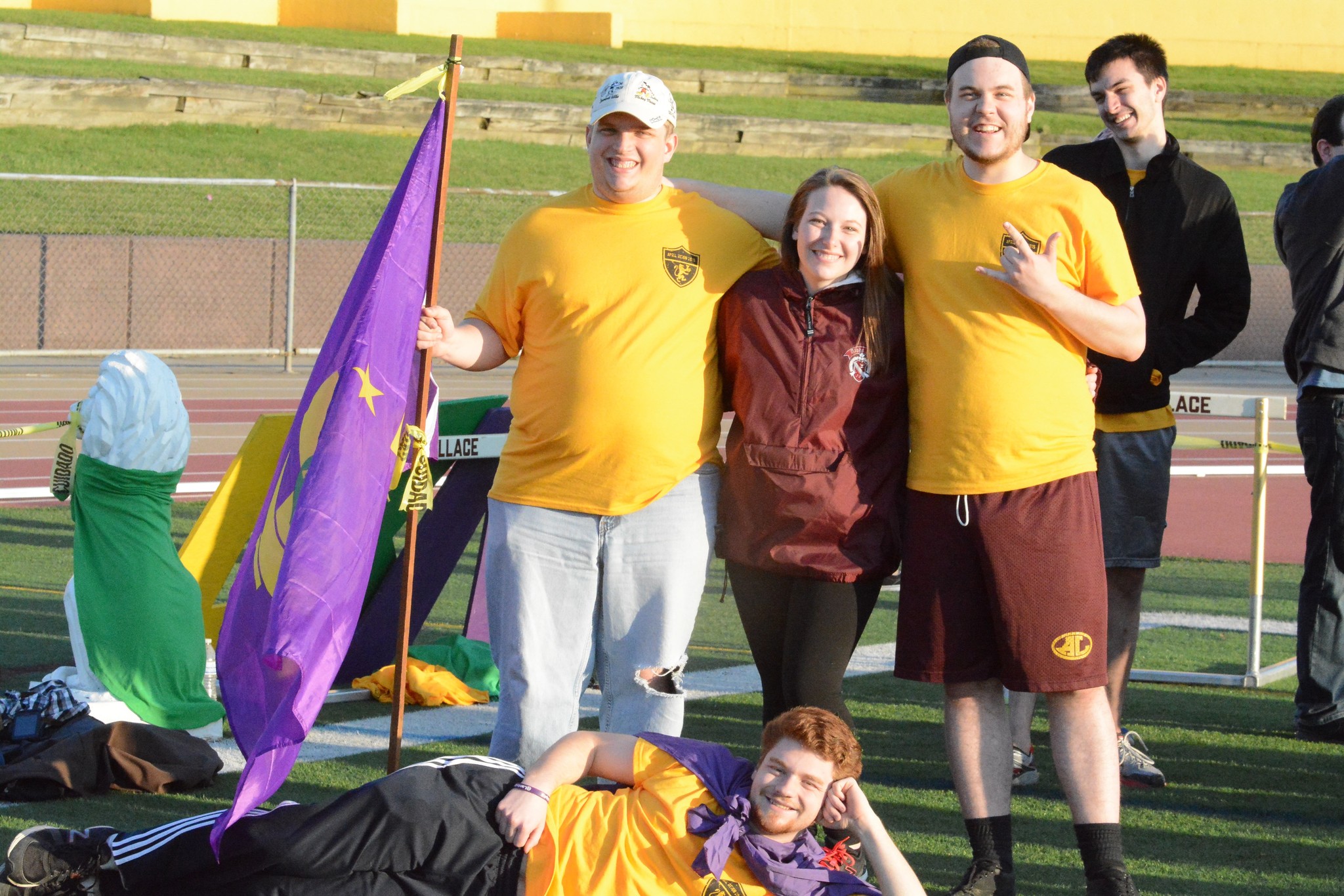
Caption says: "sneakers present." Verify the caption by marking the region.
[951,858,1015,896]
[1012,744,1041,787]
[1118,727,1165,789]
[0,861,99,896]
[818,836,867,881]
[1086,870,1139,896]
[7,825,122,887]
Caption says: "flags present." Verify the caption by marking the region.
[205,94,447,847]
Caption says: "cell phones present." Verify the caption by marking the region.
[11,712,40,741]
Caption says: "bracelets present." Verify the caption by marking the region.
[513,783,551,803]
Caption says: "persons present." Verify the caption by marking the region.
[1006,33,1251,794]
[668,34,1148,895]
[714,166,914,776]
[418,75,781,778]
[0,705,926,896]
[1271,92,1344,744]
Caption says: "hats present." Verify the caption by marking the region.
[947,35,1034,141]
[589,70,678,128]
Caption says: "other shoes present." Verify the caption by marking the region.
[1298,717,1344,744]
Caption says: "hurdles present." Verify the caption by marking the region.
[1127,390,1299,688]
